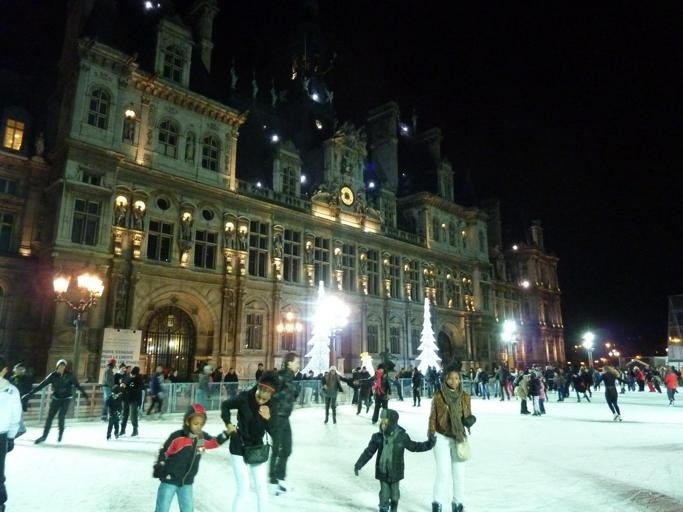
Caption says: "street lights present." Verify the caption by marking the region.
[582,332,593,368]
[578,348,619,372]
[500,319,517,370]
[315,294,349,372]
[275,307,303,354]
[52,264,103,419]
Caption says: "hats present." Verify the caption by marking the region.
[378,408,398,435]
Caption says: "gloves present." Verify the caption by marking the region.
[464,415,476,428]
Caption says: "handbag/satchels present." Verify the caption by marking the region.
[242,444,270,463]
[458,439,470,459]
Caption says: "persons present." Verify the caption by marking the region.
[426,368,474,512]
[195,361,240,400]
[221,354,304,512]
[305,362,441,424]
[102,358,178,441]
[471,362,682,422]
[352,409,438,512]
[1,354,88,511]
[152,403,236,512]
